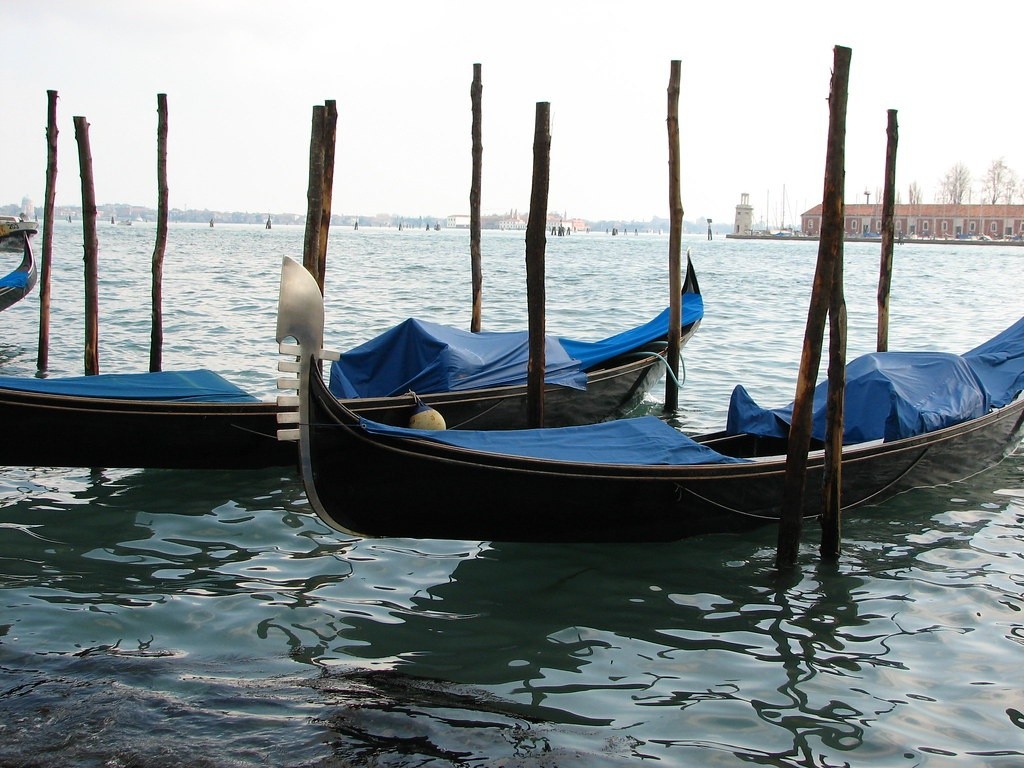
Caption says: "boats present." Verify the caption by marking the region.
[275,252,1024,545]
[0,230,38,312]
[0,213,39,244]
[0,249,708,471]
[117,219,132,225]
